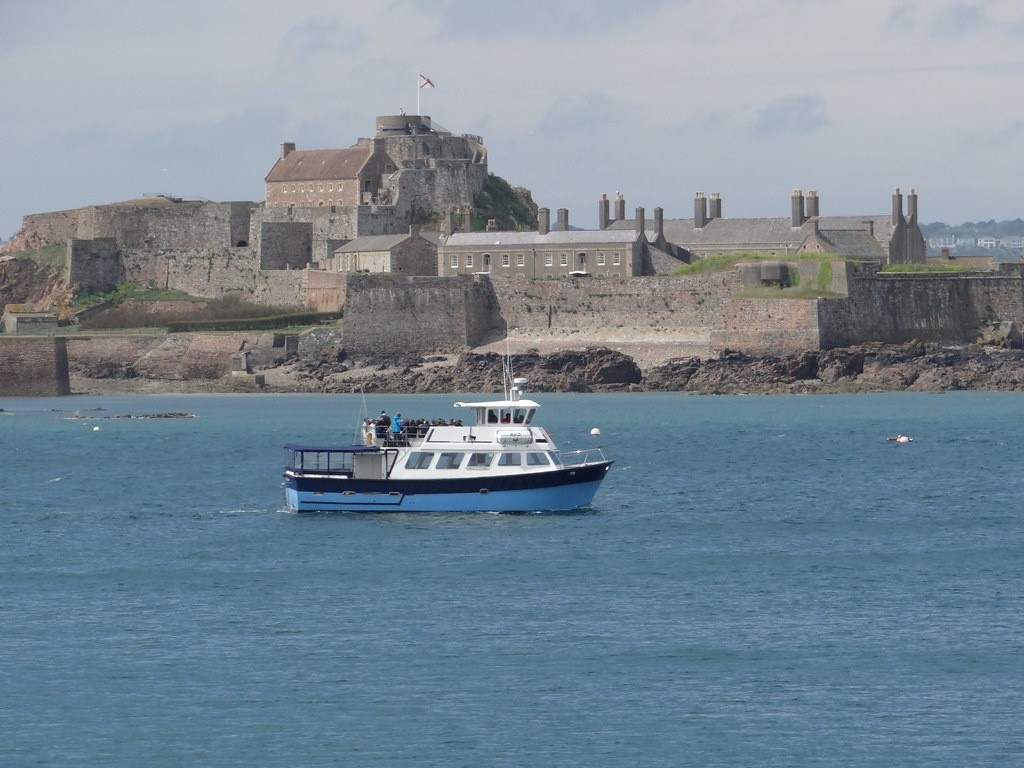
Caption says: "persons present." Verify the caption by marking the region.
[401,417,431,447]
[430,418,448,426]
[374,410,396,447]
[487,410,498,423]
[501,413,510,423]
[392,413,405,446]
[514,414,530,424]
[450,418,462,427]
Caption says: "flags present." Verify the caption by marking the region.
[417,74,435,89]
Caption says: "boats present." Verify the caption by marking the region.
[282,314,617,517]
